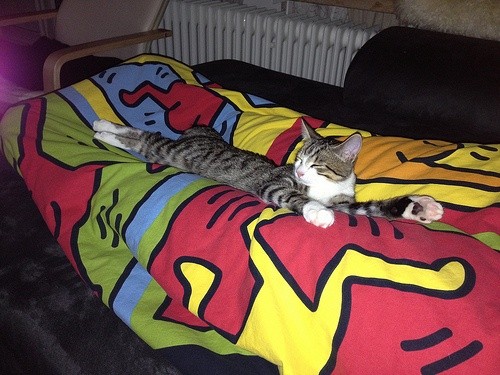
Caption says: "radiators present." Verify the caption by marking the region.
[149,0,379,87]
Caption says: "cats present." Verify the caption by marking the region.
[93,115,444,228]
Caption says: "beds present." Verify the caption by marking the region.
[0,25,500,375]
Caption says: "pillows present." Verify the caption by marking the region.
[393,0,500,40]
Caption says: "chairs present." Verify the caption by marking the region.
[0,1,173,106]
